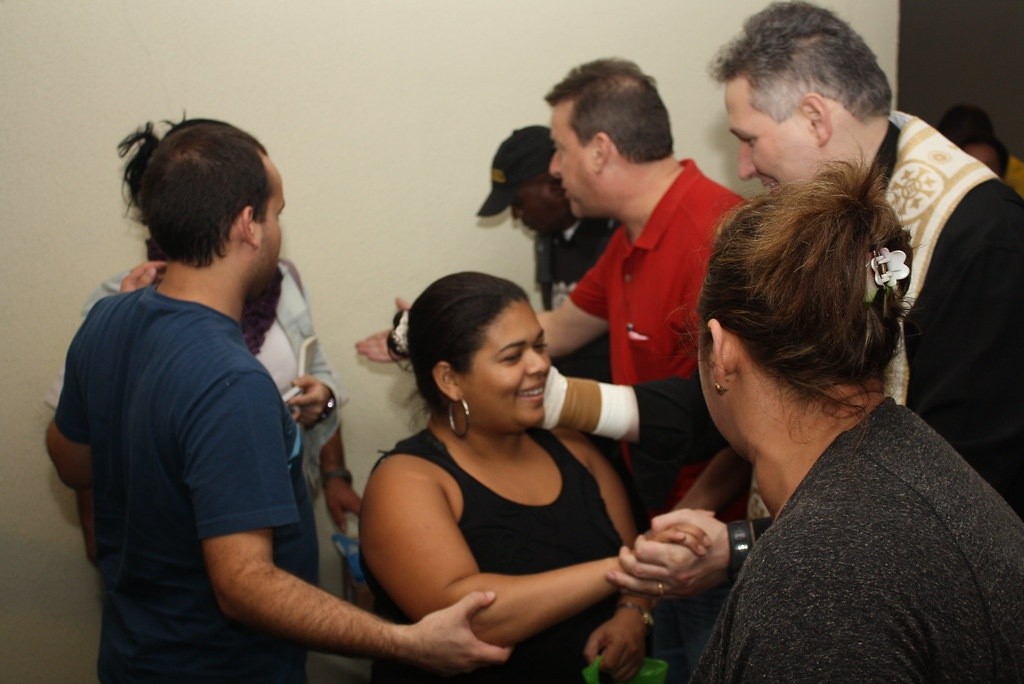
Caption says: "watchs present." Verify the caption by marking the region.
[303,389,336,429]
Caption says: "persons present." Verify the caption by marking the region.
[355,56,751,684]
[942,102,1010,177]
[45,119,514,684]
[389,1,1024,601]
[694,160,1024,684]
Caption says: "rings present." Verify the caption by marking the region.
[657,581,665,595]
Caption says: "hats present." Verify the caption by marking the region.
[477,126,557,217]
[936,105,1010,169]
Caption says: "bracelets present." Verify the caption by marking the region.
[324,469,351,484]
[616,601,654,630]
[726,519,753,585]
